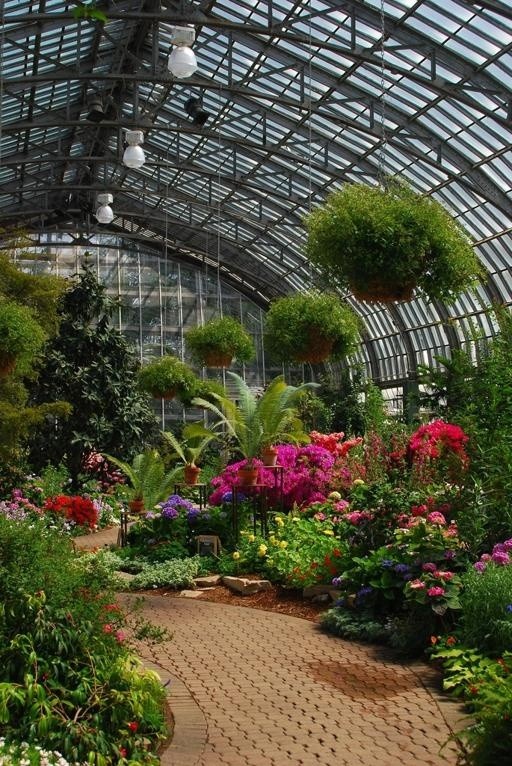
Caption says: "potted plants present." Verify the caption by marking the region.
[307,175,488,314]
[134,317,254,401]
[97,373,321,510]
[260,290,360,368]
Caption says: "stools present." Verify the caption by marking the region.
[119,513,145,548]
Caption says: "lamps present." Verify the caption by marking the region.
[88,24,208,227]
